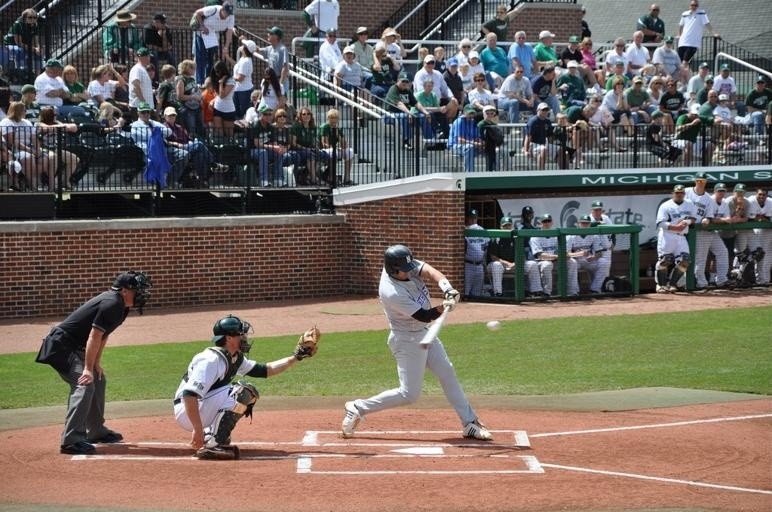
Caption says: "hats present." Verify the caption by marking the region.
[318,26,768,121]
[465,170,754,227]
[20,0,288,118]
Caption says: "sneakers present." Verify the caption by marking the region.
[462,417,493,441]
[655,280,680,296]
[60,440,97,455]
[197,444,236,460]
[7,163,359,192]
[87,430,124,444]
[342,400,362,439]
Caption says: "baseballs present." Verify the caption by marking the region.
[487,321,500,332]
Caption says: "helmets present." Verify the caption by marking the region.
[210,313,249,344]
[384,244,421,274]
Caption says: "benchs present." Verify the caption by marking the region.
[481,257,597,301]
[2,39,772,191]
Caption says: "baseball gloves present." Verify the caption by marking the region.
[296,328,320,360]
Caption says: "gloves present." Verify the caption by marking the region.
[441,298,456,312]
[444,286,461,304]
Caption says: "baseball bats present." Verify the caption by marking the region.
[420,305,451,350]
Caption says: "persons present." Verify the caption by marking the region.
[340,242,490,442]
[172,313,318,460]
[34,265,155,451]
